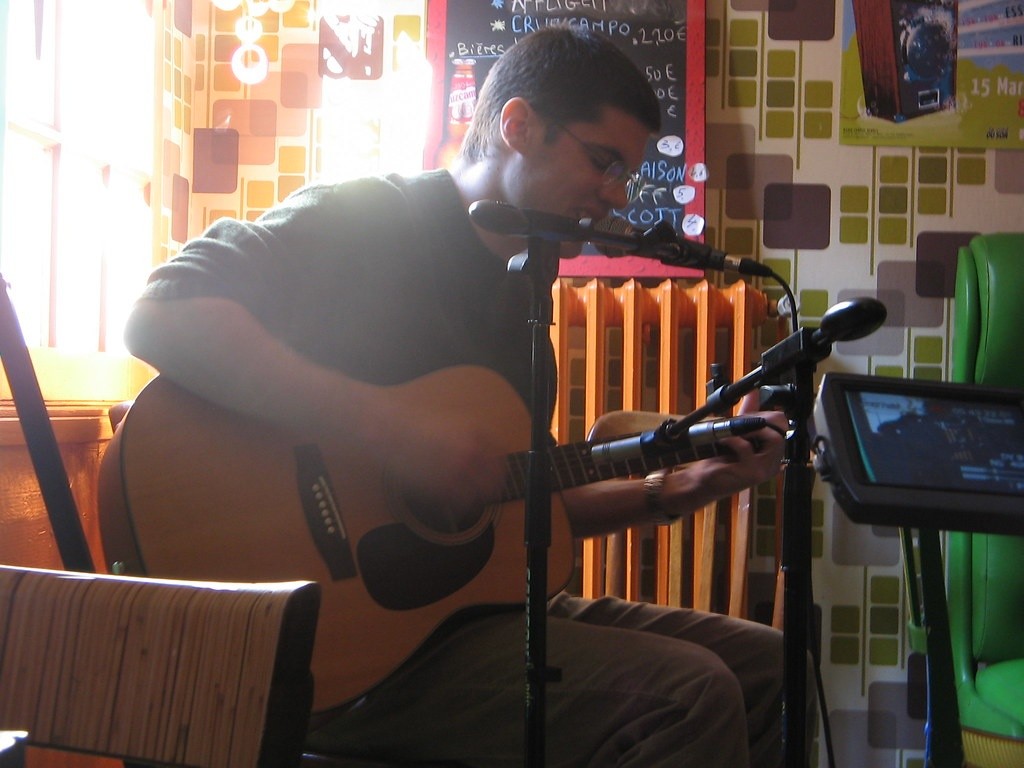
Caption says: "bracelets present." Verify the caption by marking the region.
[644,469,683,526]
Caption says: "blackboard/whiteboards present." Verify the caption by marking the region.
[425,1,705,279]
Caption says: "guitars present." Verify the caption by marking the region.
[93,368,800,715]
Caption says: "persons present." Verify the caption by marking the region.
[127,30,813,768]
[875,399,999,488]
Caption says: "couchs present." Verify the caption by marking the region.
[942,233,1024,768]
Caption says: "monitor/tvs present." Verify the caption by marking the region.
[842,383,1024,496]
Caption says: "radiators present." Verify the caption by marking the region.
[544,276,792,627]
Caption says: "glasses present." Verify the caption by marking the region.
[557,118,645,204]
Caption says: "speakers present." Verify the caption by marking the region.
[851,0,959,124]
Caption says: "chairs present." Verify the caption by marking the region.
[0,406,325,768]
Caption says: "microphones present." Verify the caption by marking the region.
[591,216,772,278]
[590,417,767,465]
